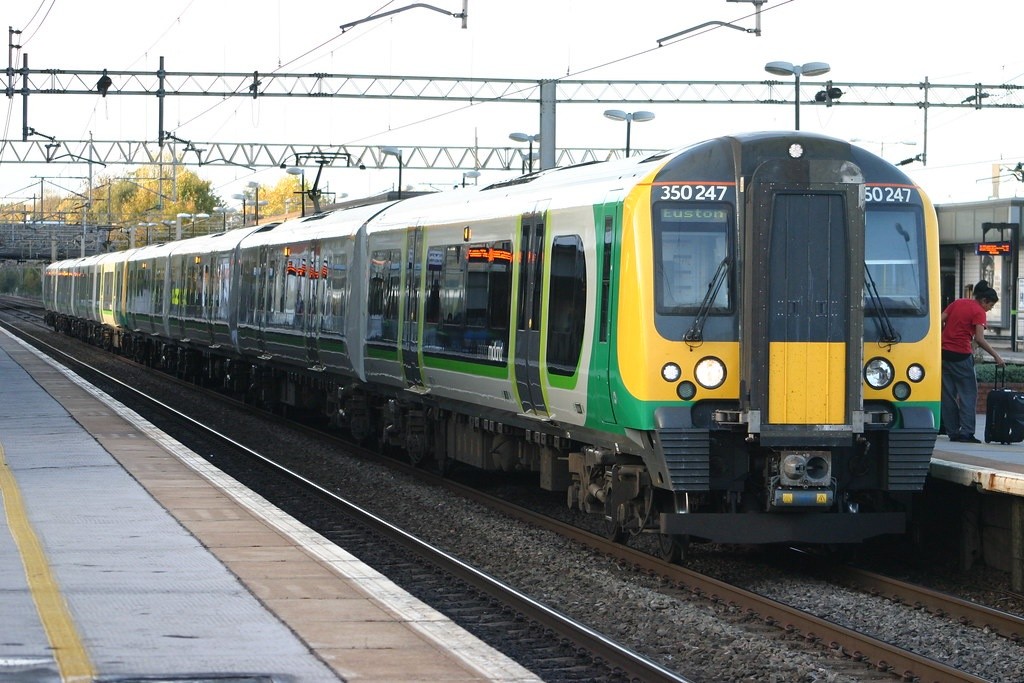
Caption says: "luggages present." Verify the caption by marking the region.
[984,364,1024,445]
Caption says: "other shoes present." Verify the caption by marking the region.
[959,435,981,443]
[950,435,959,441]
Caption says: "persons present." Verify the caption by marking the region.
[941,280,1005,443]
[982,256,994,288]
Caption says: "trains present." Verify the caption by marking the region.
[41,129,943,565]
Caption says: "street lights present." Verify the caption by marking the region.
[160,219,176,241]
[231,193,247,228]
[138,222,158,246]
[120,227,137,249]
[509,132,541,173]
[850,137,917,158]
[212,206,236,232]
[285,167,305,217]
[602,108,656,157]
[893,222,920,297]
[380,145,403,200]
[245,181,259,226]
[177,212,210,237]
[763,60,832,131]
[245,199,269,220]
[462,171,481,188]
